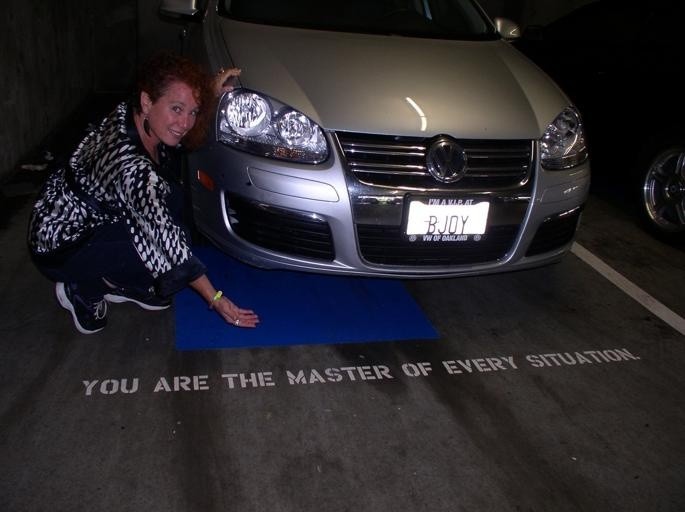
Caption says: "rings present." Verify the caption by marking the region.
[218,68,225,76]
[235,319,240,327]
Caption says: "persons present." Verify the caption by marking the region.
[27,62,261,334]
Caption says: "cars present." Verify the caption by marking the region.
[160,0,593,281]
[510,0,684,250]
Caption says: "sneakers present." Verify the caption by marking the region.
[53,281,107,335]
[103,283,173,311]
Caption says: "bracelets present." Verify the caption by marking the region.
[207,289,224,310]
